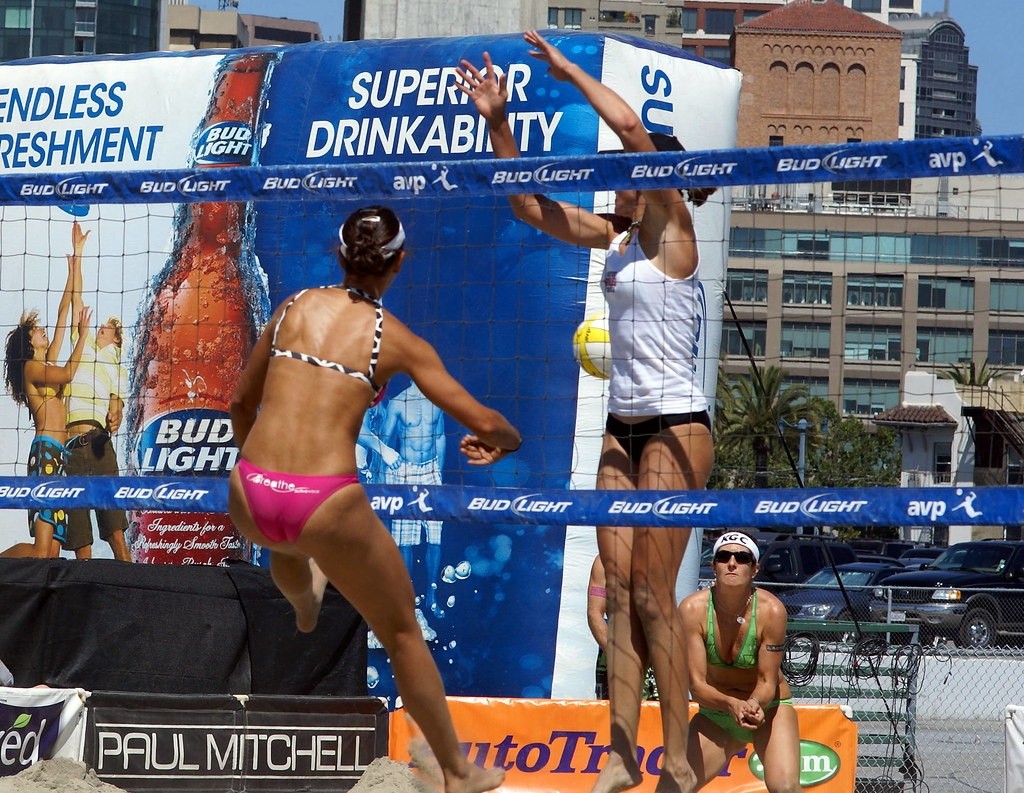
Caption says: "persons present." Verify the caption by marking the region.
[679,531,804,793]
[454,29,716,793]
[229,207,523,793]
[587,555,659,701]
[0,221,131,563]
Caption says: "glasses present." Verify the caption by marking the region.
[714,550,754,565]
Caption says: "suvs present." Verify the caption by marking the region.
[868,537,1024,653]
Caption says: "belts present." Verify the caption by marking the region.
[66,428,103,449]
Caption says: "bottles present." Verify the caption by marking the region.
[131,54,270,565]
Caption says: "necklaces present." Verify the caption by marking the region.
[626,219,645,245]
[717,594,753,617]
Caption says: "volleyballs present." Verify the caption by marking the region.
[573,312,612,378]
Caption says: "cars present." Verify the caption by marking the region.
[699,526,986,625]
[777,561,921,645]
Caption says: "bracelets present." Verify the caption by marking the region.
[501,437,523,453]
